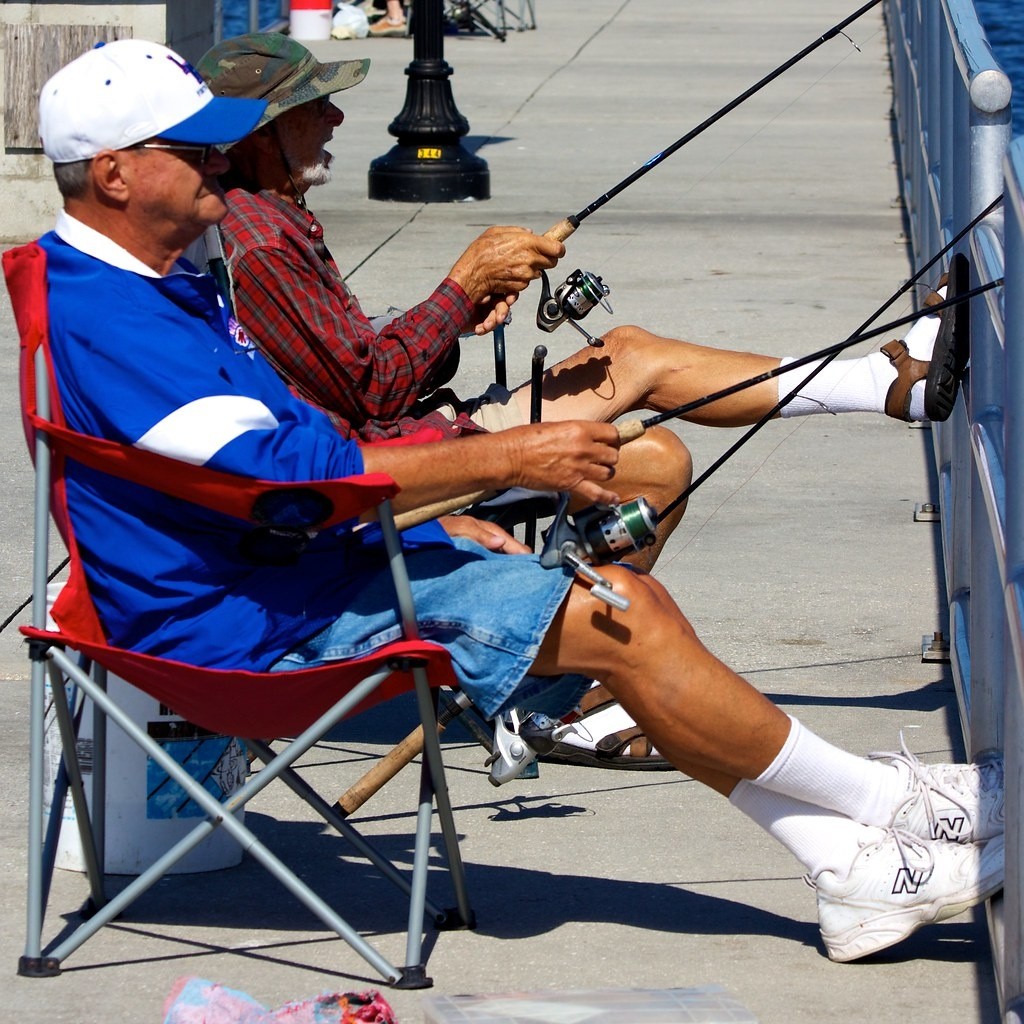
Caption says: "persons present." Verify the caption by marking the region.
[37,38,1008,961]
[192,32,968,768]
[368,0,413,38]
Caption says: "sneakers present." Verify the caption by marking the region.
[808,760,1005,964]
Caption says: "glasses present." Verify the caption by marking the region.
[133,144,214,166]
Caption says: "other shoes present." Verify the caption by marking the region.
[370,16,407,39]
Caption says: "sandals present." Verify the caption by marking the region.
[522,683,675,771]
[880,252,971,425]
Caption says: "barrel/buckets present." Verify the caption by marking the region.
[41,581,247,873]
[289,0,332,39]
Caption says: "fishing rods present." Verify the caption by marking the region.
[535,1,882,348]
[390,274,1000,616]
[330,191,1002,824]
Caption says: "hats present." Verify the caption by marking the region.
[39,40,268,164]
[195,31,373,154]
